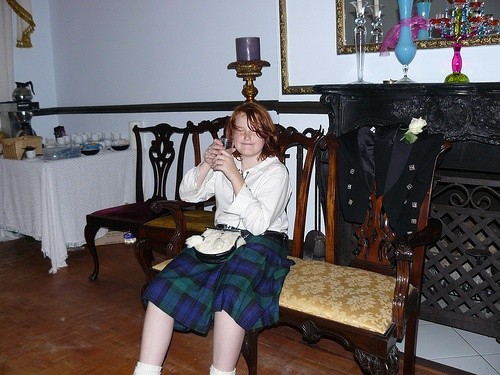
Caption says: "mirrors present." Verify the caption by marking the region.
[335,0,500,55]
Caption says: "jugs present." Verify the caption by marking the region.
[125,120,144,149]
[12,80,36,102]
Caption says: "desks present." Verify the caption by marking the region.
[0,147,154,274]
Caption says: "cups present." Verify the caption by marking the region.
[26,150,36,159]
[219,137,232,155]
[56,131,121,145]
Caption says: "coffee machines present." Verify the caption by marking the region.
[0,101,40,136]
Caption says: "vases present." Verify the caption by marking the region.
[393,0,420,84]
[417,2,431,38]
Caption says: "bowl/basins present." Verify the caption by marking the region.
[81,149,101,156]
[109,145,129,151]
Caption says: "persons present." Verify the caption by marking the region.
[134,103,296,375]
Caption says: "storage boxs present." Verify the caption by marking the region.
[0,133,44,160]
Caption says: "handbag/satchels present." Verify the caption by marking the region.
[186,225,248,264]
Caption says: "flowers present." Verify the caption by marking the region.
[400,116,426,145]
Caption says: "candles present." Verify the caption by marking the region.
[234,36,261,60]
[356,0,362,10]
[374,0,380,13]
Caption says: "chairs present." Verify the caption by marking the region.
[84,116,452,375]
